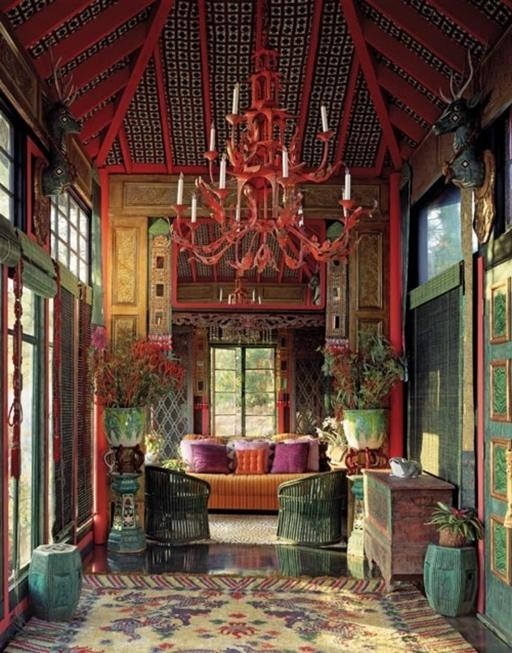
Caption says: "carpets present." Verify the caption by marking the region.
[1,567,478,653]
[143,506,351,553]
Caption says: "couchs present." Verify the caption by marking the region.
[174,432,329,514]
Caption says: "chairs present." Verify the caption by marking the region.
[273,468,353,548]
[144,461,214,547]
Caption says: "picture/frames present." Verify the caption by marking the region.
[489,354,511,427]
[486,274,510,345]
[486,436,512,505]
[485,511,511,588]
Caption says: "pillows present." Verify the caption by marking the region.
[178,432,321,475]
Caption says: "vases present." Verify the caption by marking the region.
[101,404,147,449]
[340,406,390,447]
[329,447,350,464]
[145,451,163,464]
[437,526,466,547]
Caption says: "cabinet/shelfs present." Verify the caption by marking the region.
[357,463,457,600]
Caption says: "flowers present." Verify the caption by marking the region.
[316,414,350,446]
[144,431,166,453]
[84,317,185,406]
[422,499,486,542]
[316,324,407,408]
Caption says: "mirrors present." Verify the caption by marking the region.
[171,214,327,310]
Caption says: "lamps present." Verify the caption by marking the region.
[162,14,371,276]
[215,278,263,304]
